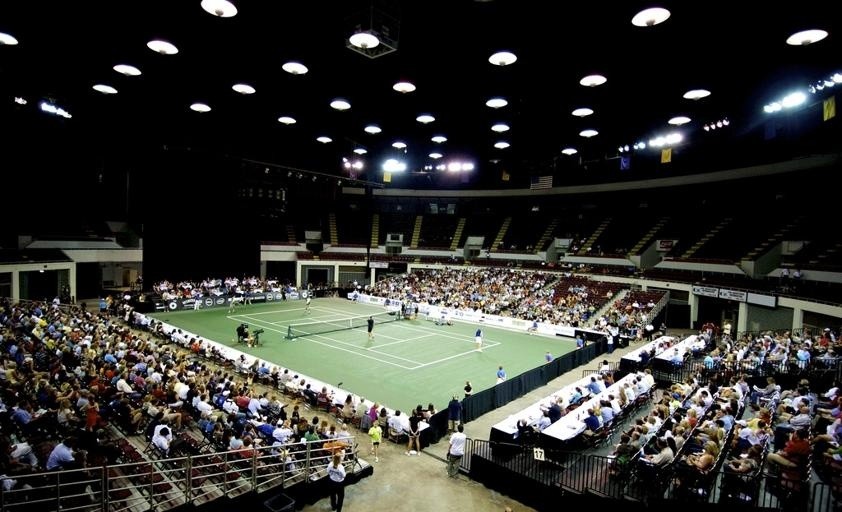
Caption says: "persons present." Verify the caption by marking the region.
[304,295,312,314]
[258,275,348,303]
[0,274,258,512]
[348,239,657,319]
[326,453,346,512]
[496,366,507,385]
[258,360,473,482]
[780,267,792,278]
[474,327,483,353]
[367,316,375,339]
[518,318,842,512]
[792,268,805,278]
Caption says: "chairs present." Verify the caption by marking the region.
[0,269,842,512]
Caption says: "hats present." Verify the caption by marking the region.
[222,389,231,396]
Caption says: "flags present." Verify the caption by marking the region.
[620,156,631,170]
[350,168,358,180]
[501,169,511,181]
[383,171,392,184]
[822,95,839,122]
[661,148,673,164]
[764,117,776,140]
[461,176,469,184]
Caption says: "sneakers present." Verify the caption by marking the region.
[406,450,422,457]
[371,450,380,464]
